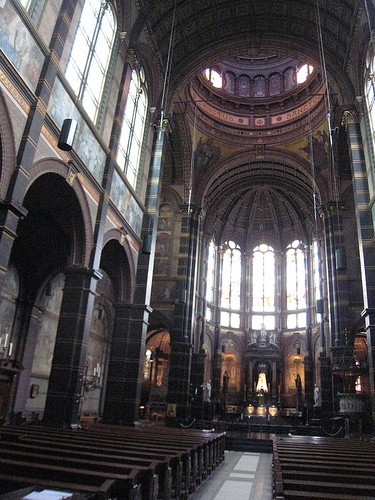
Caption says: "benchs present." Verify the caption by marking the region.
[0,422,375,500]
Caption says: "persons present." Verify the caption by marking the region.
[294,373,304,396]
[206,380,212,403]
[313,384,321,407]
[222,371,229,392]
